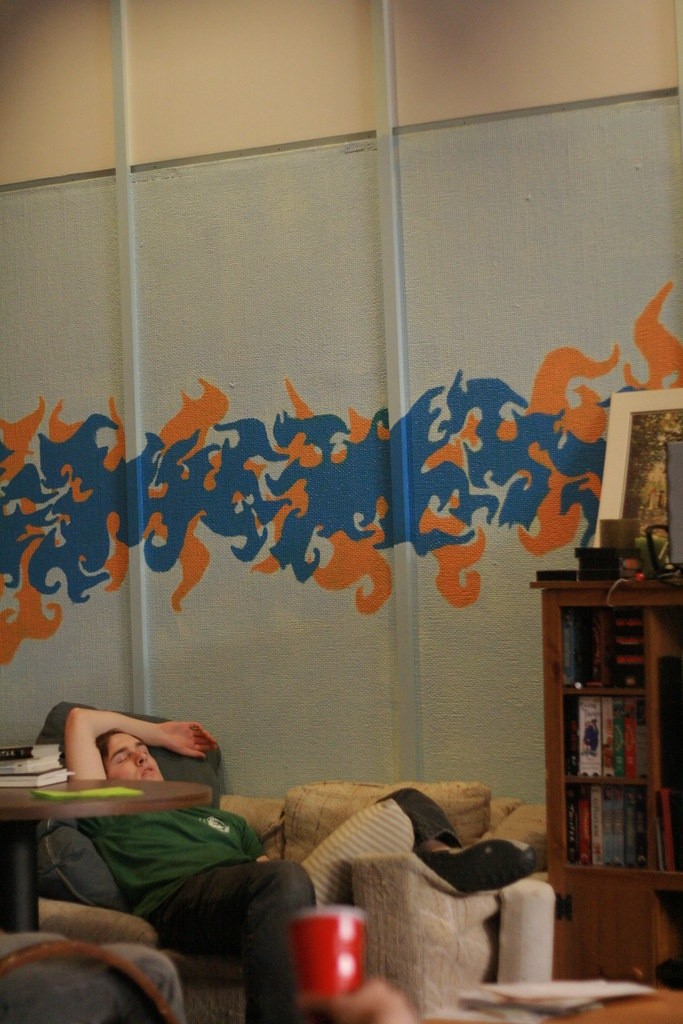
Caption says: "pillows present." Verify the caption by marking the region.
[302,791,448,905]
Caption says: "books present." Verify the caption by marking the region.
[562,607,649,870]
[0,744,67,788]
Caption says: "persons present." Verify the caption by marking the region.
[0,927,186,1024]
[64,707,535,1024]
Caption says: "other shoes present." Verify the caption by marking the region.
[418,838,538,894]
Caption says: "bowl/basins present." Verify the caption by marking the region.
[574,547,640,580]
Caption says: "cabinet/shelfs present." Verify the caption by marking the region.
[529,569,683,992]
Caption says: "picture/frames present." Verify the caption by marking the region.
[591,387,683,570]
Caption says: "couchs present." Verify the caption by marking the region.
[0,778,554,1024]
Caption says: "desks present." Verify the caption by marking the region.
[0,779,215,934]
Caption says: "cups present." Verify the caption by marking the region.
[600,519,640,547]
[636,539,667,577]
[290,905,368,995]
